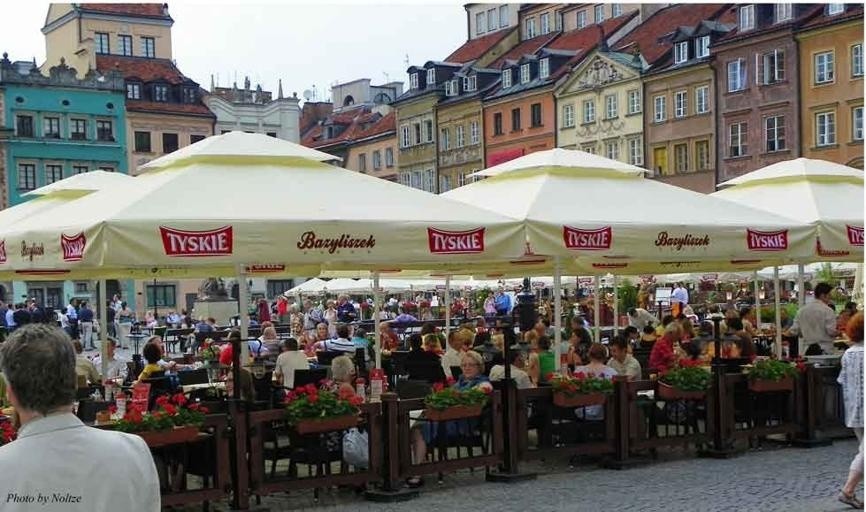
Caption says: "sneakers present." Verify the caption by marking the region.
[837,489,863,509]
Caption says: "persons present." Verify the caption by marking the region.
[165,310,195,328]
[106,293,131,350]
[70,336,197,382]
[191,315,217,354]
[295,294,376,382]
[201,321,310,382]
[1,296,98,351]
[376,285,556,383]
[558,281,862,382]
[248,293,297,323]
[145,310,159,330]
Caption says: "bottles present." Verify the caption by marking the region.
[782,342,790,359]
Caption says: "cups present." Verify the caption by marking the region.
[115,392,126,417]
[355,377,367,405]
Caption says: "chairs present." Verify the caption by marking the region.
[0,280,864,511]
